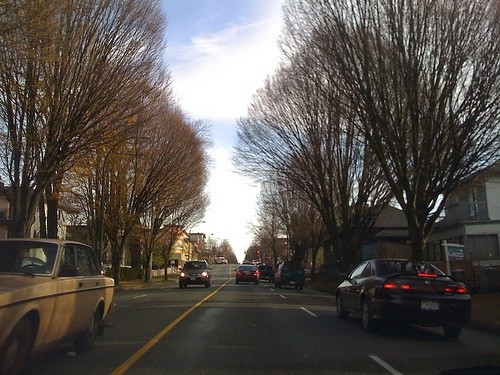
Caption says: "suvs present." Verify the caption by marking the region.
[179,260,212,289]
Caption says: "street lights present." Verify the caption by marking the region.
[188,221,205,260]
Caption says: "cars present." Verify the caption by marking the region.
[216,256,228,264]
[0,238,114,375]
[234,260,305,290]
[335,258,472,339]
